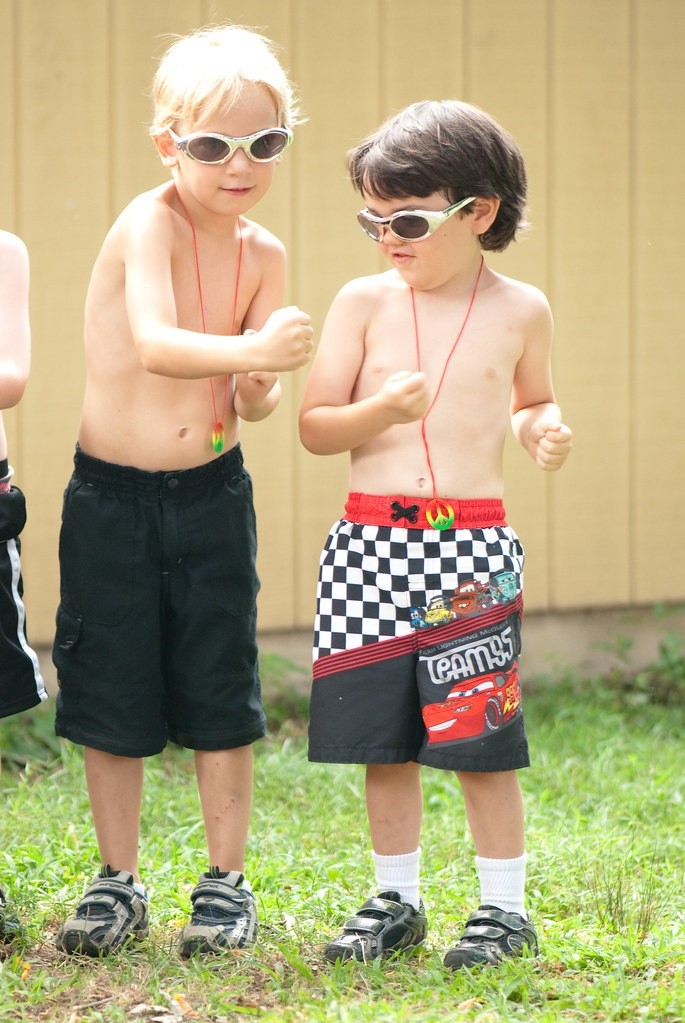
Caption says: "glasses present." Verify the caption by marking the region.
[357,195,476,242]
[167,122,294,165]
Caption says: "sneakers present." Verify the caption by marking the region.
[324,891,428,966]
[177,866,258,957]
[444,905,539,971]
[56,864,149,960]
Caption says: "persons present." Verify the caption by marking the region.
[298,96,573,975]
[51,22,313,965]
[0,226,49,965]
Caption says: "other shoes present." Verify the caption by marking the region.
[0,889,22,962]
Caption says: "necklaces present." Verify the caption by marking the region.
[409,253,485,531]
[171,180,243,453]
